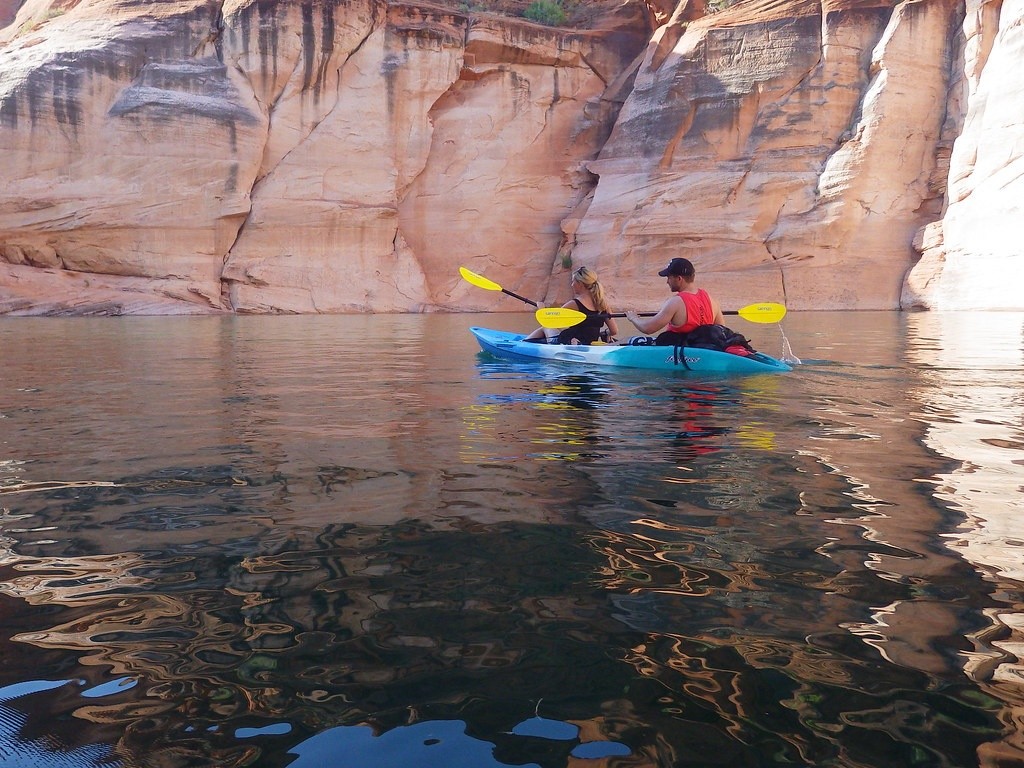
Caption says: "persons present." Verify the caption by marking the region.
[571,258,725,346]
[524,265,618,345]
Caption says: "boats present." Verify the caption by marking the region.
[470,322,796,374]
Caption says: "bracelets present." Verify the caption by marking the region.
[604,328,609,336]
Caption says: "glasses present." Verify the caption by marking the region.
[578,267,585,276]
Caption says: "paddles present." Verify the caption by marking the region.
[534,302,789,330]
[458,266,540,309]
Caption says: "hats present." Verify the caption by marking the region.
[659,258,693,277]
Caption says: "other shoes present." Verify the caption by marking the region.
[568,337,582,345]
